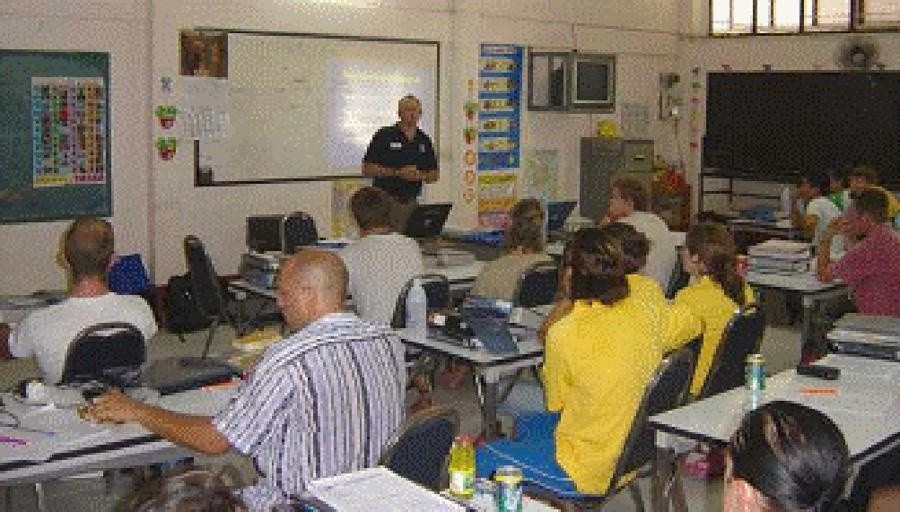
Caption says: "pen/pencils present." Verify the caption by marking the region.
[799,389,836,393]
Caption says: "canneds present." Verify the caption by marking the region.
[741,352,767,389]
[494,464,527,512]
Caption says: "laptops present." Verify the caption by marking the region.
[548,201,577,231]
[401,204,454,237]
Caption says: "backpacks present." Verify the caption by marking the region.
[165,271,213,342]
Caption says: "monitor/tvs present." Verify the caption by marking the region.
[245,212,284,254]
[550,57,615,109]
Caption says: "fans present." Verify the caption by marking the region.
[833,34,886,70]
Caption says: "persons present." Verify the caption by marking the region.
[336,187,424,325]
[609,174,675,291]
[675,225,756,398]
[605,221,666,302]
[0,214,160,383]
[90,249,407,505]
[474,228,702,498]
[469,200,555,301]
[361,95,440,231]
[108,464,252,512]
[723,401,853,511]
[790,165,900,354]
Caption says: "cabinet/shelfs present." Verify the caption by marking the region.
[579,137,655,226]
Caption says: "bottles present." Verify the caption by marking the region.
[449,432,476,501]
[407,276,427,335]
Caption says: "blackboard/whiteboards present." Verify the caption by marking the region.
[704,70,900,191]
[193,27,441,188]
